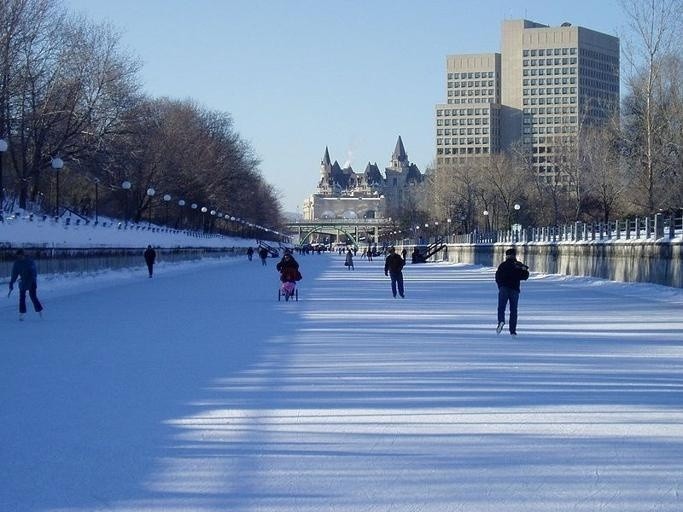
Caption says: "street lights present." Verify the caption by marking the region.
[390,210,489,239]
[0,139,8,206]
[52,155,63,220]
[121,181,294,244]
[514,202,521,224]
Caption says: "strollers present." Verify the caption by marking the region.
[279,266,299,302]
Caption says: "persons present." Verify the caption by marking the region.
[268,243,384,262]
[344,249,354,271]
[245,246,254,262]
[493,247,529,336]
[276,253,302,300]
[412,246,418,264]
[384,247,405,299]
[8,246,43,321]
[258,247,268,266]
[143,244,156,278]
[400,247,407,260]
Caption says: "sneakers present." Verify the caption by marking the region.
[496,321,504,333]
[510,332,517,335]
